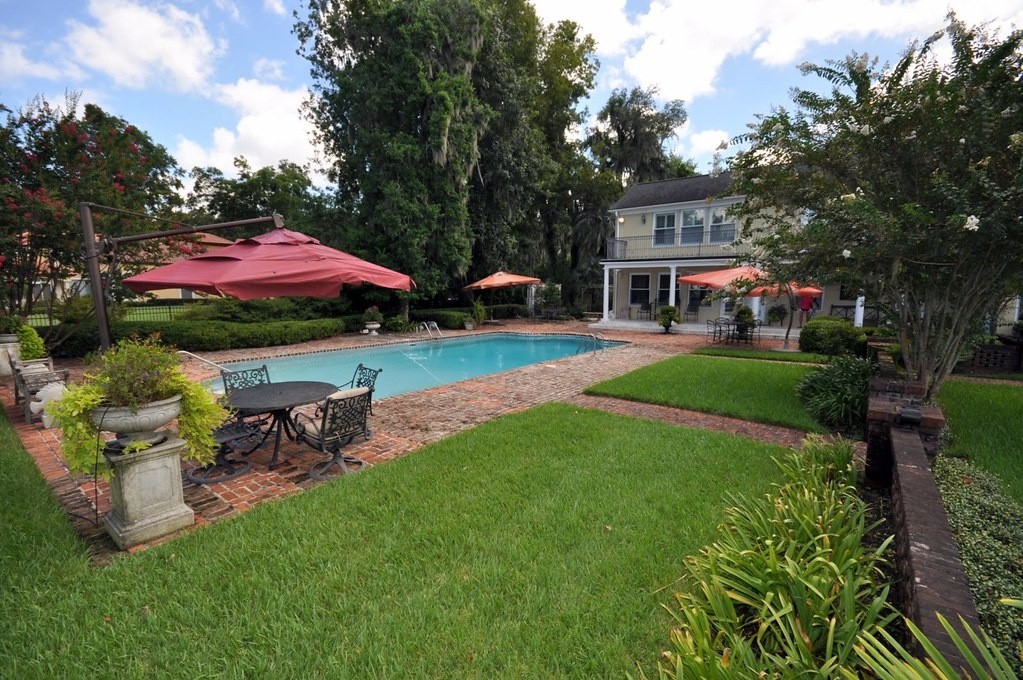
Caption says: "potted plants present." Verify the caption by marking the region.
[463,294,487,330]
[0,314,26,343]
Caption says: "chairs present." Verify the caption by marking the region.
[707,317,762,346]
[186,362,383,483]
[637,303,650,321]
[684,304,699,323]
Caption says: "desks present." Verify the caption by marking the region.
[224,381,339,469]
[724,322,745,344]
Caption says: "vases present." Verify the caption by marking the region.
[365,322,380,336]
[92,393,184,447]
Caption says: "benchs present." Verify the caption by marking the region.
[830,304,879,328]
[649,298,680,323]
[6,346,69,424]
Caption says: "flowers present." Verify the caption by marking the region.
[46,332,240,484]
[361,306,384,324]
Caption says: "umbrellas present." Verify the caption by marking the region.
[122,227,417,301]
[464,271,541,292]
[679,265,824,298]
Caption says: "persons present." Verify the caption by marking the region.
[797,295,817,328]
